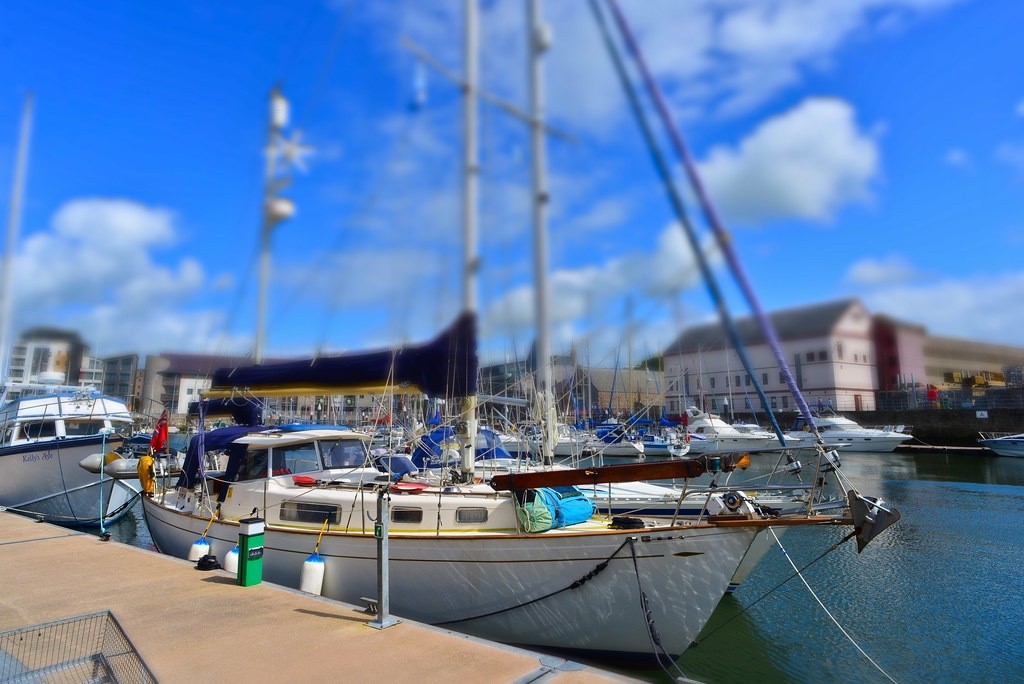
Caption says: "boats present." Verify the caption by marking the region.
[446,401,913,458]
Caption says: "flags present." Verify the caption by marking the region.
[150,410,167,454]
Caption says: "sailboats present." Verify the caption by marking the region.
[141,1,794,664]
[1,383,143,532]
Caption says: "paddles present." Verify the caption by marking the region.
[290,474,430,493]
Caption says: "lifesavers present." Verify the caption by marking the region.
[139,456,156,493]
